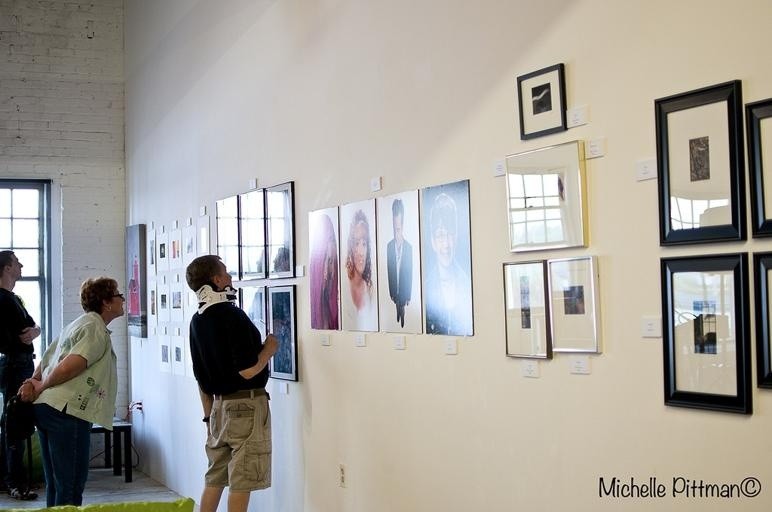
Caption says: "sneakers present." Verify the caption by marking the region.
[7,487,38,500]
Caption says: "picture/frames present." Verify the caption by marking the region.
[241,285,266,347]
[238,188,265,281]
[231,286,241,307]
[504,139,589,255]
[660,251,755,418]
[652,78,748,248]
[517,62,567,141]
[502,259,553,362]
[266,284,299,382]
[744,98,772,239]
[754,251,772,392]
[264,181,296,280]
[215,194,239,282]
[549,255,602,359]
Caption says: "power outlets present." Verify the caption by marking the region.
[136,401,144,413]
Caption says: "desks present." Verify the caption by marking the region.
[91,414,133,483]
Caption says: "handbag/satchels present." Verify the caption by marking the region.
[4,395,35,449]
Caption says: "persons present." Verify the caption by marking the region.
[311,214,339,331]
[186,257,279,511]
[341,210,378,332]
[0,251,41,499]
[387,198,413,327]
[18,277,126,506]
[424,194,471,335]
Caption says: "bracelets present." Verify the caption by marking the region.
[31,376,40,381]
[203,418,212,424]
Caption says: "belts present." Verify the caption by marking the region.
[211,388,267,400]
[0,354,37,359]
